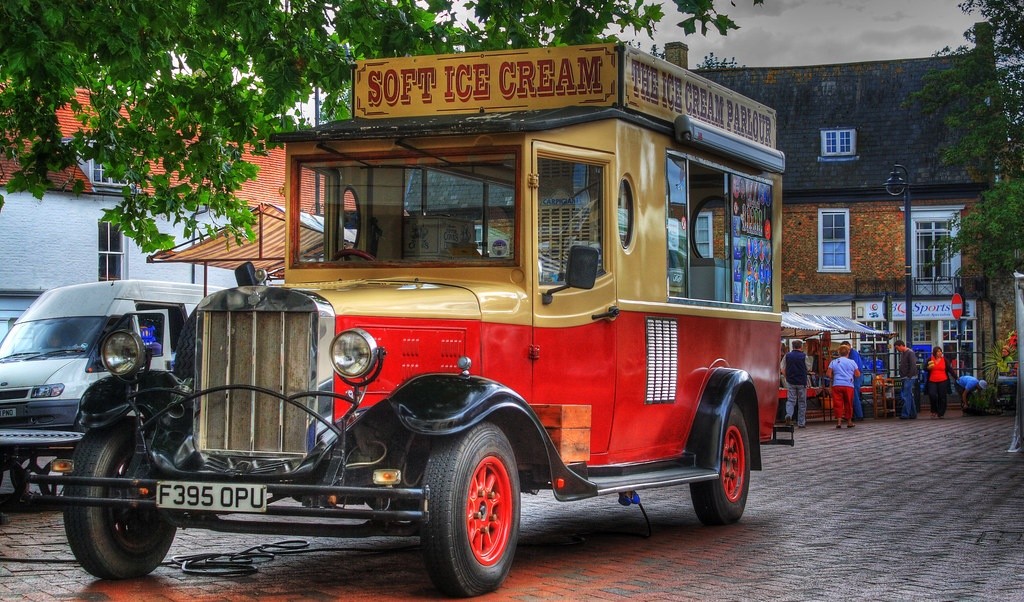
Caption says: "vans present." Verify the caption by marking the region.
[0,280,229,498]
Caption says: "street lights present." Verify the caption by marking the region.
[880,164,914,349]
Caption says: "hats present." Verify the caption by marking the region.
[978,380,988,389]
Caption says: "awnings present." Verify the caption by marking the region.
[780,312,897,339]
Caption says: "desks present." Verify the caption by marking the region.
[779,387,832,423]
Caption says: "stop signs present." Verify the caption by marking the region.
[951,293,964,320]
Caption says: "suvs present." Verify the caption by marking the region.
[21,40,796,602]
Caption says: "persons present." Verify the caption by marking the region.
[997,345,1014,375]
[954,376,987,417]
[923,346,960,419]
[894,340,917,420]
[826,341,864,428]
[780,340,812,428]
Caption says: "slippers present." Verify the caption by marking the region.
[836,423,841,428]
[847,423,855,428]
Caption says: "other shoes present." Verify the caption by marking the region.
[851,416,864,421]
[909,415,917,419]
[899,415,909,419]
[938,414,944,419]
[785,415,791,426]
[931,412,936,419]
[798,424,806,428]
[963,412,968,417]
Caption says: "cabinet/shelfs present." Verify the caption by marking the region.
[402,217,476,261]
[873,378,896,418]
[689,258,726,302]
